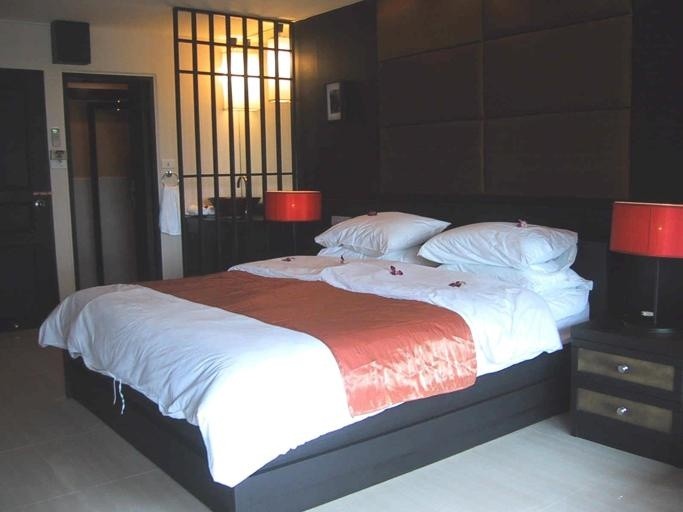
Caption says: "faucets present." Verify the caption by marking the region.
[236,176,247,189]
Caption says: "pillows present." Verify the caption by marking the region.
[314,212,591,292]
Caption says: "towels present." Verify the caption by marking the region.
[157,182,182,236]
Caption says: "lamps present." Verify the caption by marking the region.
[608,200,683,333]
[263,190,322,256]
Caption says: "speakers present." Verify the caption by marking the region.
[50,21,90,65]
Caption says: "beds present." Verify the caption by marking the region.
[61,194,615,512]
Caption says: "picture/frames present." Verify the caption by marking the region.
[323,80,344,122]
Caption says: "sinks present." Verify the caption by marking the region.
[208,196,261,215]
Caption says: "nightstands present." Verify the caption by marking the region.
[570,313,682,469]
